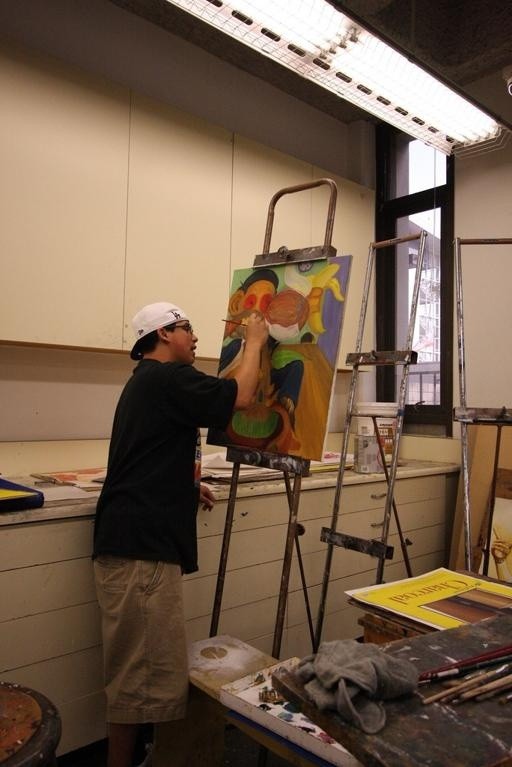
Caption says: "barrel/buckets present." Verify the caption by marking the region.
[356,399,401,473]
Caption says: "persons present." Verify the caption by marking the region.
[91,302,270,767]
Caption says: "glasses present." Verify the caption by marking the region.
[163,323,190,332]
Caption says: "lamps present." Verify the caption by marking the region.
[162,0,512,161]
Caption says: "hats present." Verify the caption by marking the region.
[130,302,189,360]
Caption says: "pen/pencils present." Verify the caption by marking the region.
[419,645,512,705]
[221,319,248,326]
